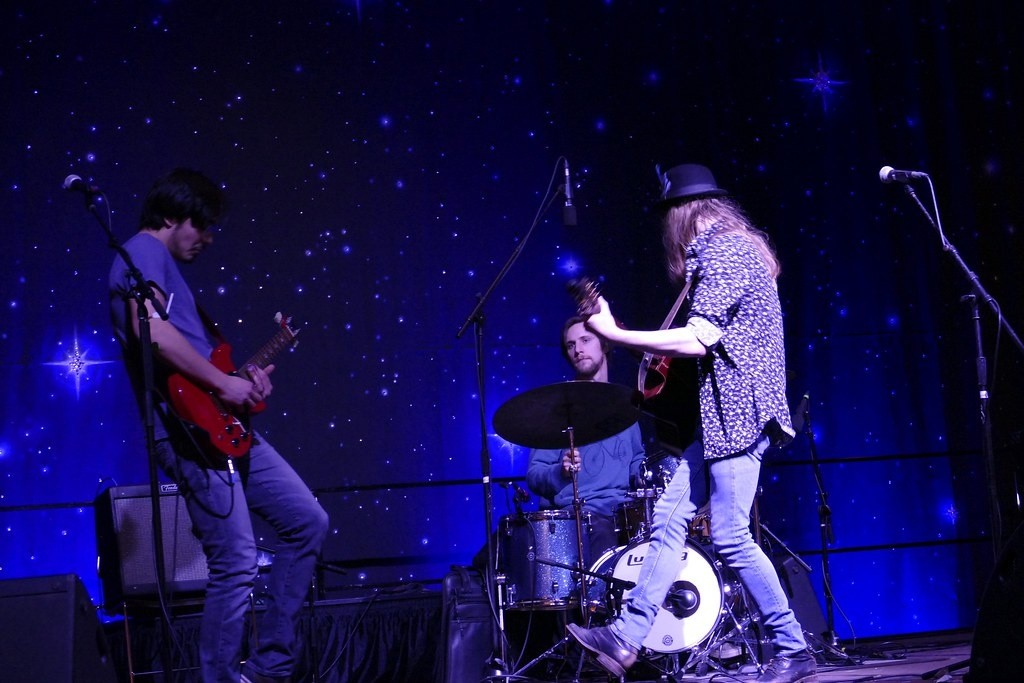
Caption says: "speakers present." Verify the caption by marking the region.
[772,554,832,650]
[0,573,118,683]
[94,481,211,609]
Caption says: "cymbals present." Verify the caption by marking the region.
[491,376,643,448]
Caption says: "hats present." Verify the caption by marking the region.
[652,164,729,206]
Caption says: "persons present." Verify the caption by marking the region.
[524,315,652,619]
[103,168,331,683]
[567,160,819,683]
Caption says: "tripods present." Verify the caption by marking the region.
[505,424,678,683]
[674,412,906,683]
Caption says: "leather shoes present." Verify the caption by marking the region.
[757,649,818,683]
[564,622,638,678]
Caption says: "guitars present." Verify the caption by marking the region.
[564,275,697,413]
[165,311,300,472]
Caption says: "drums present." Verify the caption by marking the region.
[498,505,619,615]
[612,494,661,545]
[584,533,727,655]
[638,448,684,488]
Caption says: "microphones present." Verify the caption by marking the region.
[513,484,530,499]
[879,166,928,184]
[562,159,572,207]
[64,174,102,196]
[792,393,811,433]
